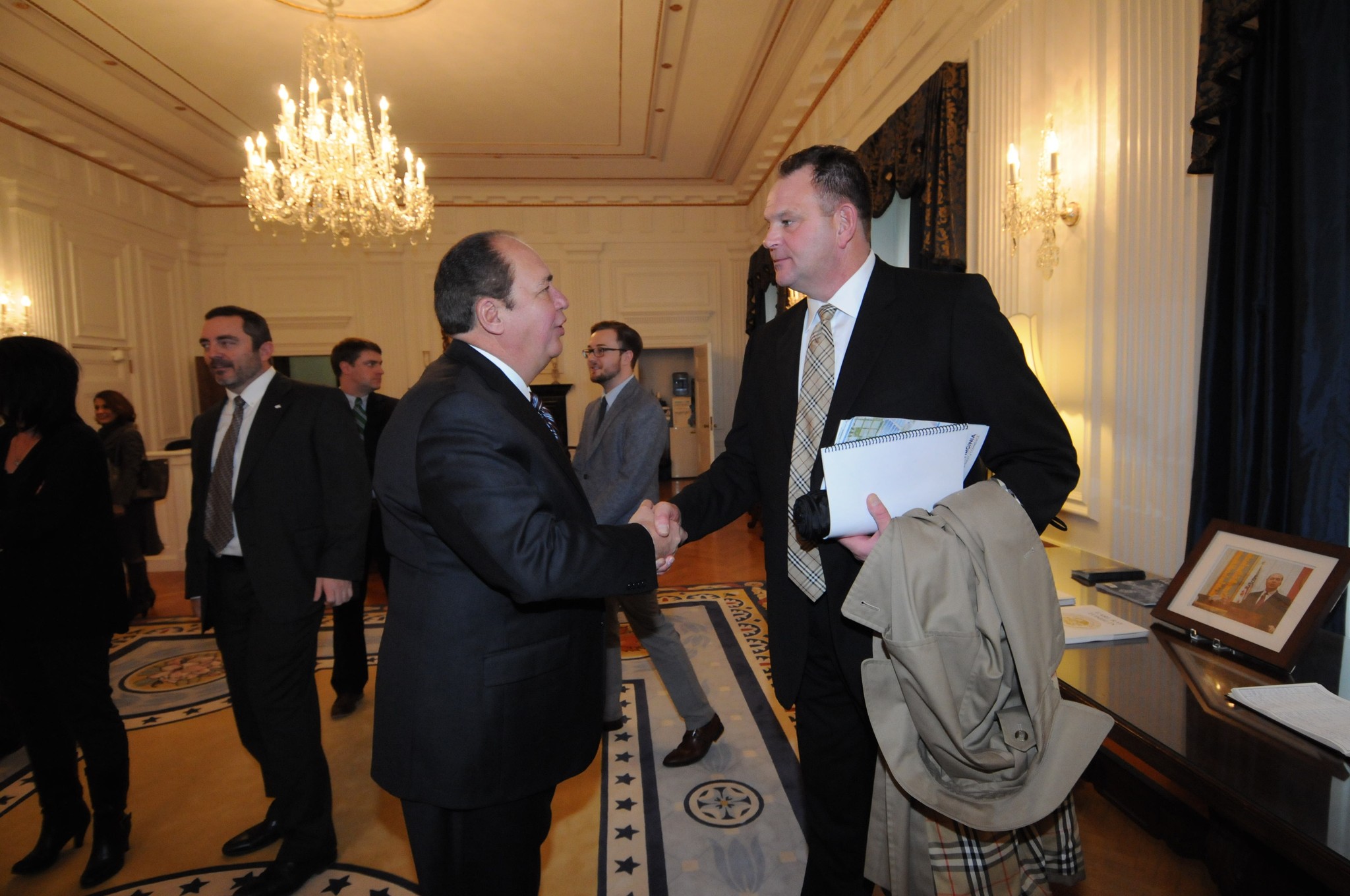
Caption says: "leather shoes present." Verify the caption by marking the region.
[222,819,289,857]
[233,849,337,895]
[330,690,364,719]
[663,712,724,767]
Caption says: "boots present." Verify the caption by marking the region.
[80,757,132,890]
[11,752,91,877]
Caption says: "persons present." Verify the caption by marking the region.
[93,388,164,633]
[0,336,133,890]
[369,227,687,895]
[649,145,1082,894]
[186,303,358,896]
[328,336,400,719]
[569,322,725,766]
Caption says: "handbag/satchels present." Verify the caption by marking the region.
[133,458,170,504]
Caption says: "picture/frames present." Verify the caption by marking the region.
[1148,513,1350,672]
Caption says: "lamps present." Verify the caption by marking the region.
[0,290,34,336]
[240,0,434,251]
[998,113,1081,280]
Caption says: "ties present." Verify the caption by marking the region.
[204,396,246,554]
[351,396,368,441]
[529,391,571,459]
[787,304,837,604]
[593,395,608,438]
[1255,592,1269,611]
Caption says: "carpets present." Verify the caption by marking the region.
[1,579,809,896]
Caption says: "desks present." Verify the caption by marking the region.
[1030,542,1349,896]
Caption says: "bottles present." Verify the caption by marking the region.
[672,371,689,396]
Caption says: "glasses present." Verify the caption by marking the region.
[582,347,627,359]
